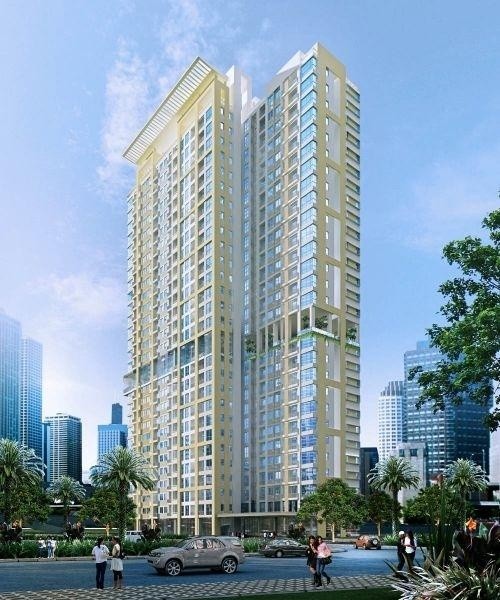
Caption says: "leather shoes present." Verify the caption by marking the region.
[112,586,122,589]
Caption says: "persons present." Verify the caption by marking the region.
[305,535,318,585]
[39,535,57,559]
[110,536,123,589]
[92,538,110,589]
[404,531,417,573]
[316,534,332,588]
[397,530,405,571]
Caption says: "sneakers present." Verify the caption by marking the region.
[97,585,104,589]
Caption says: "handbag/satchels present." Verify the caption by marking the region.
[102,551,109,558]
[319,557,332,565]
[118,553,126,560]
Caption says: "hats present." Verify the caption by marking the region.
[398,531,405,535]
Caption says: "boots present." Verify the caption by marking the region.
[311,572,321,587]
[323,574,331,585]
